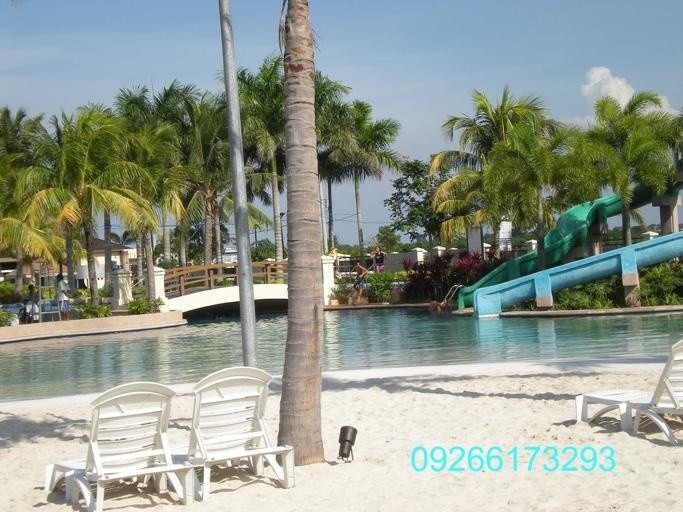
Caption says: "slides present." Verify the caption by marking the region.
[457,159,683,320]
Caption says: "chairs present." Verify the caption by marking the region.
[576,339,683,446]
[171,366,295,502]
[46,381,194,512]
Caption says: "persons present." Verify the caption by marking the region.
[55,273,71,320]
[21,284,39,323]
[348,261,368,305]
[369,245,388,272]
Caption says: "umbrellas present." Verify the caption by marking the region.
[5,255,69,279]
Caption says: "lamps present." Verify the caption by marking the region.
[337,426,358,462]
